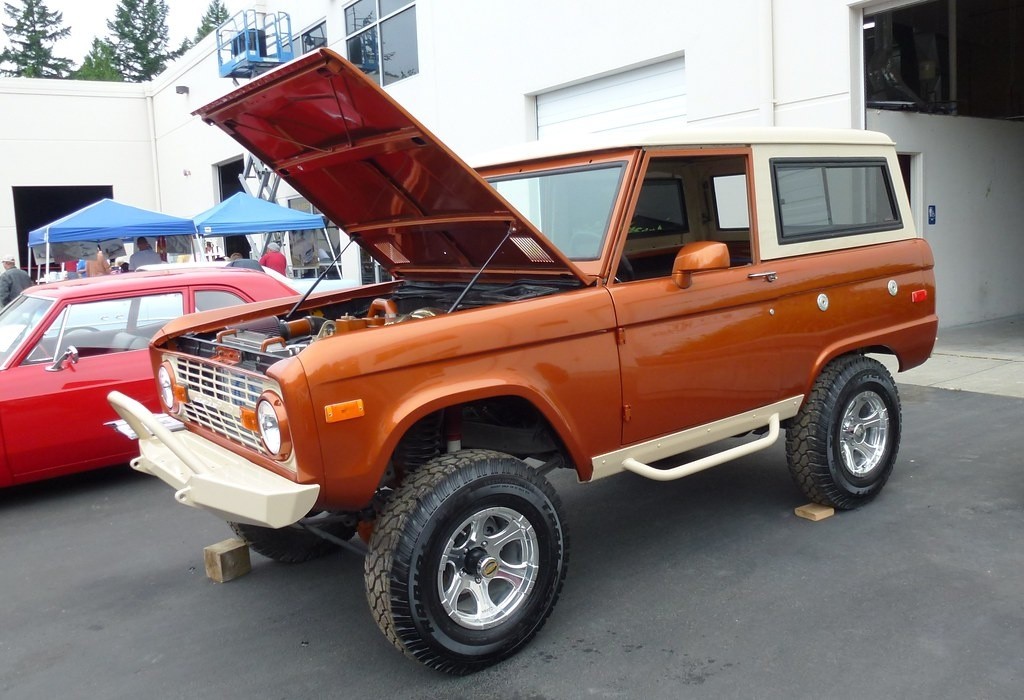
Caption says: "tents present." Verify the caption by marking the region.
[190,191,343,281]
[27,197,206,286]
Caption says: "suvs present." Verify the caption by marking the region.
[103,48,937,680]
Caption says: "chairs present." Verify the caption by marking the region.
[107,333,134,353]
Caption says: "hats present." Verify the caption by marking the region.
[267,242,280,252]
[1,256,15,263]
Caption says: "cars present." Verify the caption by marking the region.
[290,232,316,264]
[130,259,359,296]
[0,268,303,489]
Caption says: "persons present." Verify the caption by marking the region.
[0,255,34,312]
[65,236,163,279]
[224,242,288,278]
